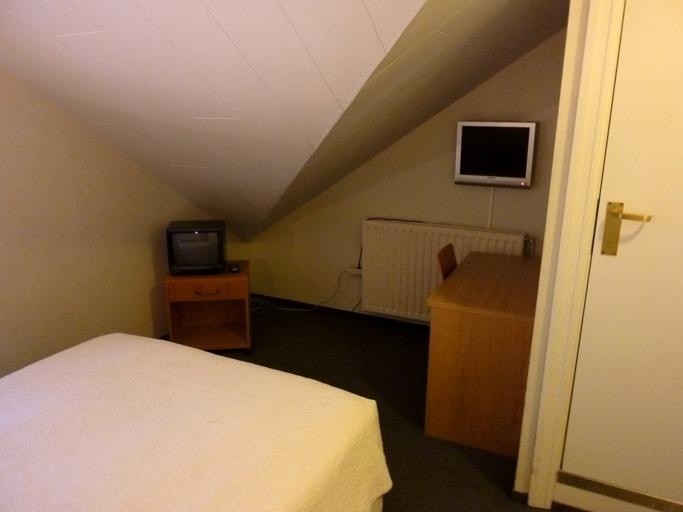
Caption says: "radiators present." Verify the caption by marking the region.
[361,217,535,323]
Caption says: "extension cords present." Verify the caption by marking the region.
[346,268,361,275]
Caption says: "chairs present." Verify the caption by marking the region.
[436,242,458,280]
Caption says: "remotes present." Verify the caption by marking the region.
[230,263,239,273]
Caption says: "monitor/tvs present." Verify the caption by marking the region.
[454,121,537,189]
[166,220,225,276]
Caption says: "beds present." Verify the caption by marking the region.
[0,332,394,512]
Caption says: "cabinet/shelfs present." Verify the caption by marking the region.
[164,260,253,354]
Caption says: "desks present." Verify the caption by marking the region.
[423,251,541,458]
